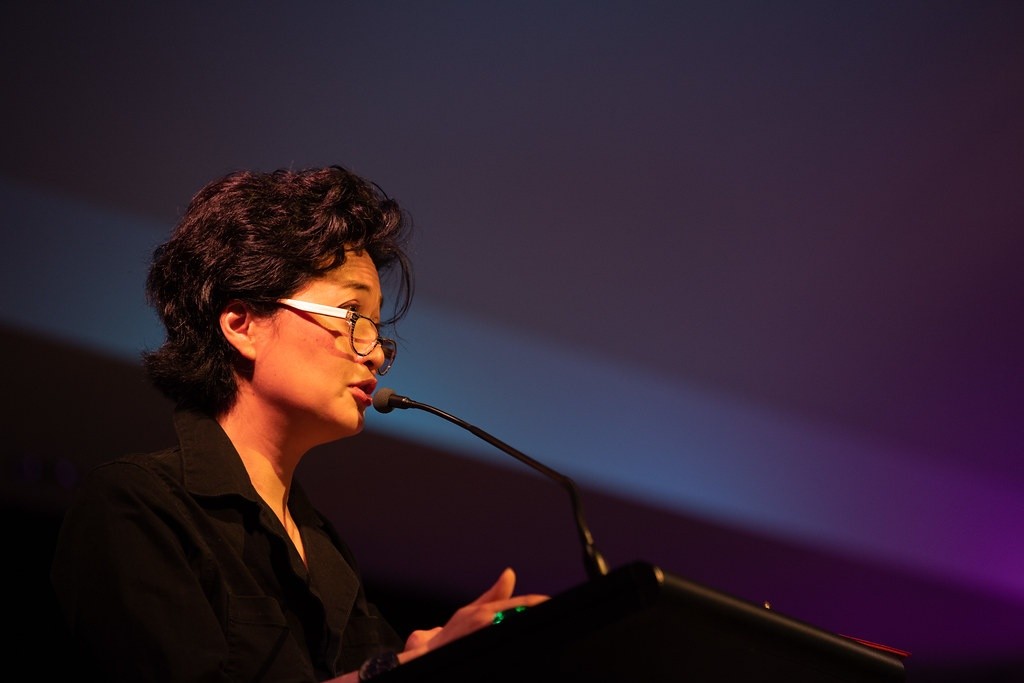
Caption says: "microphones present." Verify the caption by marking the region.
[372,388,609,581]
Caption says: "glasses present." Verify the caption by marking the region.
[249,295,397,376]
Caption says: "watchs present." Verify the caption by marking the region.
[359,651,399,683]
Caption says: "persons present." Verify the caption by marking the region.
[49,166,550,683]
